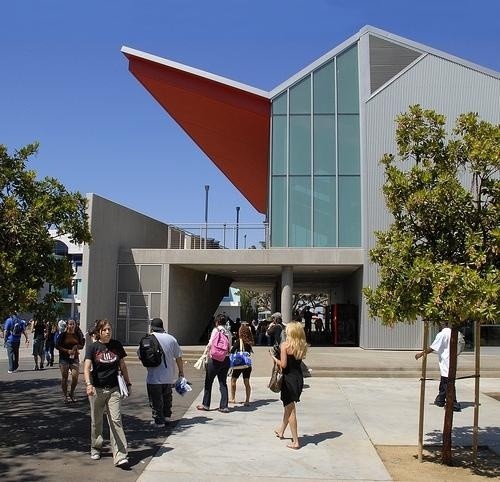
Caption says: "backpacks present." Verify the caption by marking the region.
[11,318,22,337]
[209,327,230,363]
[139,334,168,369]
[75,327,85,350]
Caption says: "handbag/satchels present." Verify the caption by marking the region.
[54,332,59,346]
[268,372,283,393]
[230,352,251,371]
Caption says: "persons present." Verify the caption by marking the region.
[0,308,135,467]
[291,303,341,344]
[136,315,188,431]
[268,319,308,450]
[195,310,285,413]
[414,319,465,414]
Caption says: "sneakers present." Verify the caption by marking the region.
[152,417,169,428]
[64,393,78,404]
[7,365,19,375]
[115,459,128,467]
[91,455,100,460]
[434,400,461,412]
[34,359,53,371]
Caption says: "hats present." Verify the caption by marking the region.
[270,312,282,319]
[151,318,164,331]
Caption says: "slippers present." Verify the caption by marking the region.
[288,444,300,450]
[196,404,207,411]
[274,430,284,439]
[220,408,229,414]
